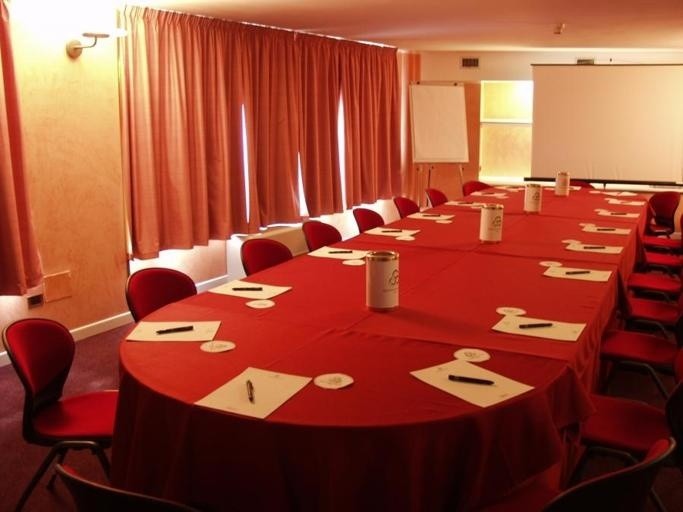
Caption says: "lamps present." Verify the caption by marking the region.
[66,17,109,59]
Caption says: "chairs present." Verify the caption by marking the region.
[539,437,676,512]
[462,180,491,196]
[55,462,185,512]
[600,329,678,399]
[626,271,682,293]
[124,267,197,323]
[645,191,680,239]
[393,196,420,218]
[644,250,682,268]
[619,294,682,338]
[1,316,120,512]
[240,237,294,278]
[353,208,384,234]
[641,214,682,250]
[425,188,448,208]
[302,221,342,252]
[570,381,682,512]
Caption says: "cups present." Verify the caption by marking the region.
[554,172,569,197]
[364,250,400,311]
[478,203,504,244]
[523,184,541,214]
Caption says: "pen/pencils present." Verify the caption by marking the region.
[597,228,616,231]
[458,202,474,205]
[156,325,193,333]
[518,322,552,328]
[422,214,439,217]
[615,192,624,197]
[232,287,262,290]
[481,193,495,196]
[565,270,589,273]
[245,379,253,401]
[506,186,519,190]
[621,202,633,204]
[583,246,605,249]
[448,373,494,386]
[328,250,352,253]
[381,230,402,232]
[610,212,626,215]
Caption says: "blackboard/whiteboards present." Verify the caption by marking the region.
[409,80,469,164]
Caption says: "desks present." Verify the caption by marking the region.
[119,183,648,512]
